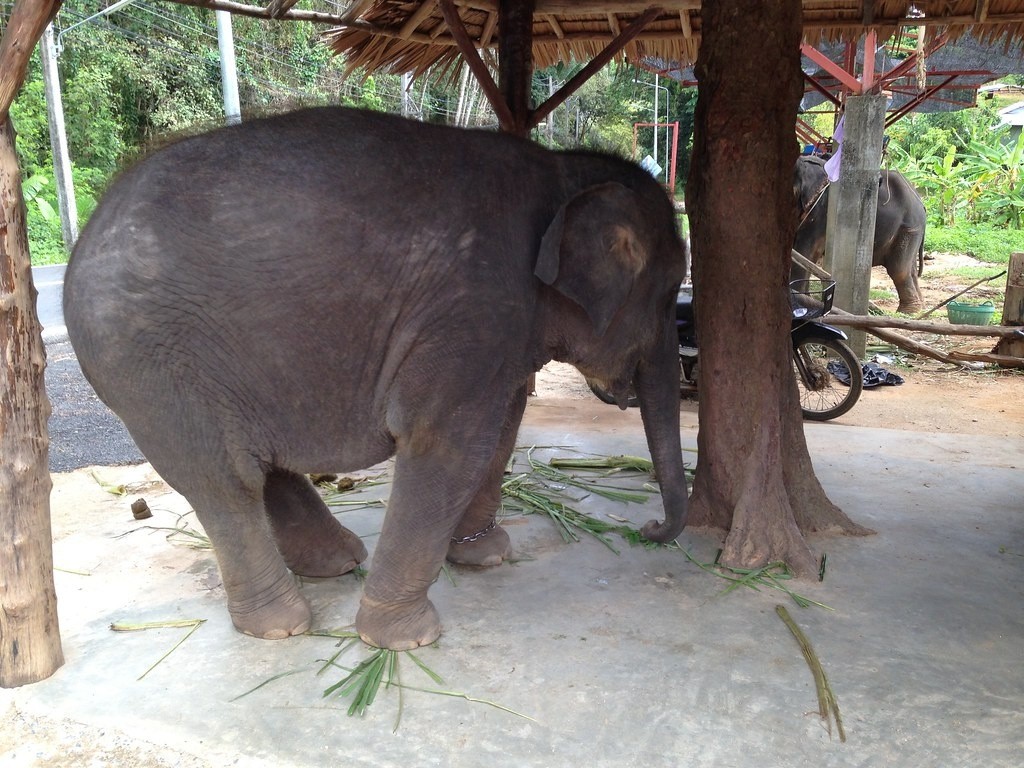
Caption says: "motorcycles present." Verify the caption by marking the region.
[582,277,865,421]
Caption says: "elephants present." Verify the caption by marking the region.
[790,156,927,317]
[64,105,687,651]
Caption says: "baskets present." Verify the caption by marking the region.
[792,278,836,319]
[946,299,995,325]
